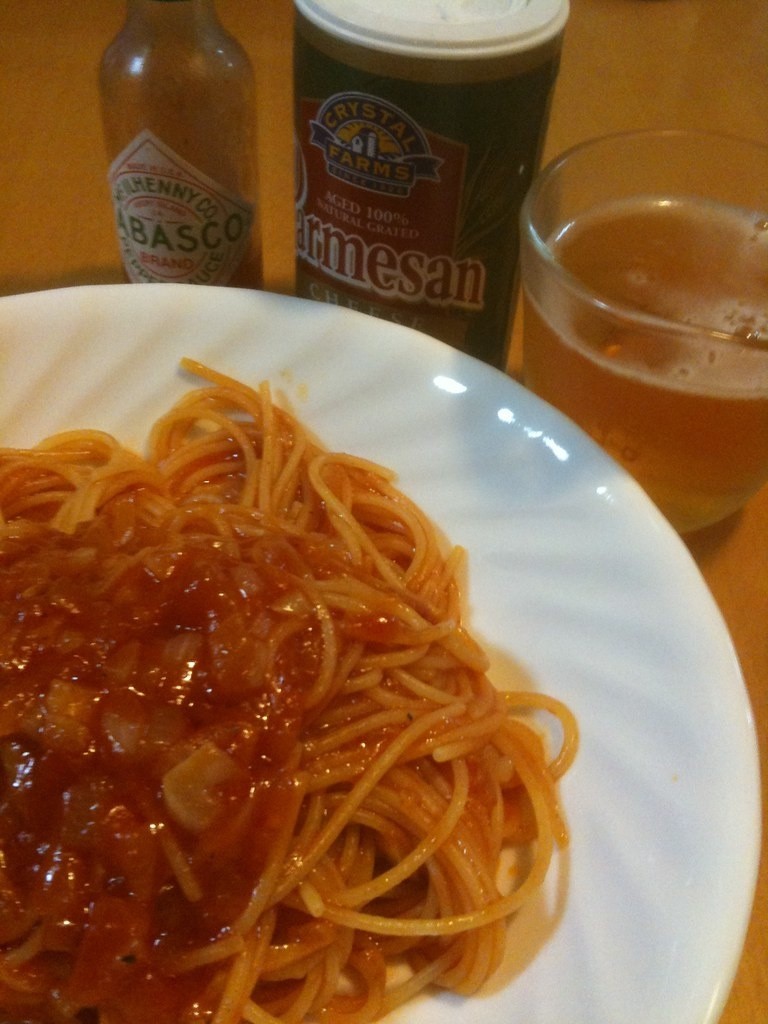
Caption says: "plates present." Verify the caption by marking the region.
[0,284,762,1023]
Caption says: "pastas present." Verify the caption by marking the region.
[0,359,580,1024]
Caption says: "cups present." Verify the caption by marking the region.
[520,126,767,536]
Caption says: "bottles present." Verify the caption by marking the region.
[96,0,263,291]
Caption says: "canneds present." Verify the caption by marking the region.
[289,0,570,380]
